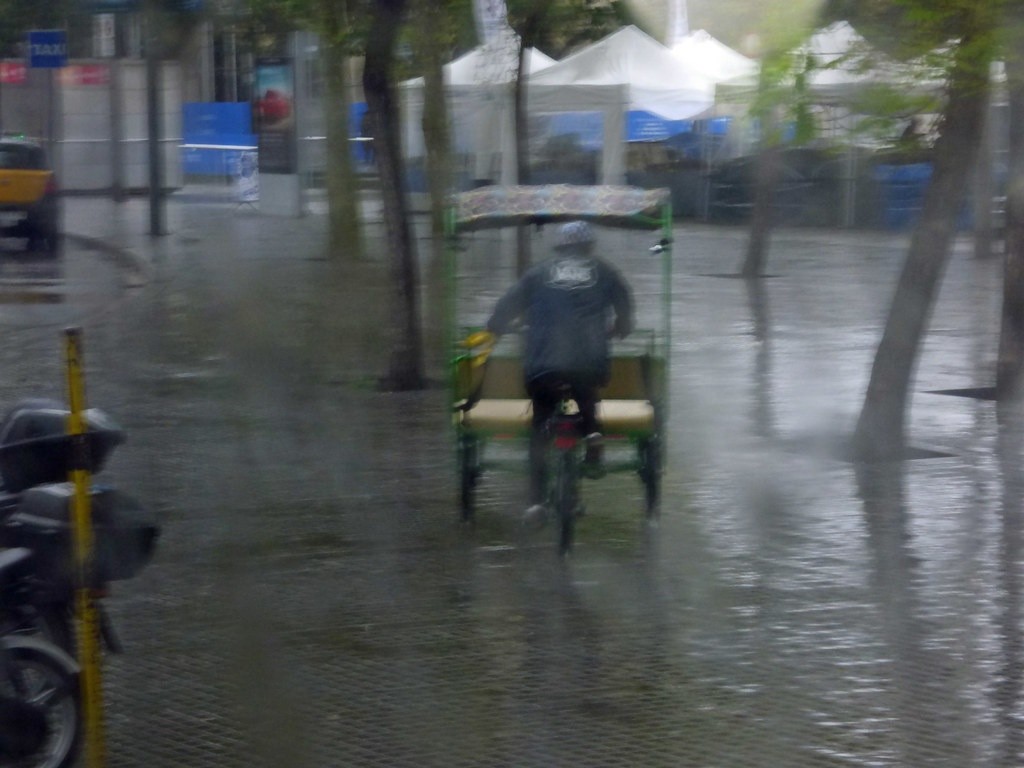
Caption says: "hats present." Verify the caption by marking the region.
[551,220,600,246]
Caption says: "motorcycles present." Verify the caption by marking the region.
[0,399,160,768]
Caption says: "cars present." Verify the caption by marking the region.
[2,136,54,240]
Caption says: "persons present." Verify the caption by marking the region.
[487,219,637,507]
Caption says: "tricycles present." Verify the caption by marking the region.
[439,184,675,546]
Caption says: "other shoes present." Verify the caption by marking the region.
[522,504,549,526]
[582,433,606,475]
